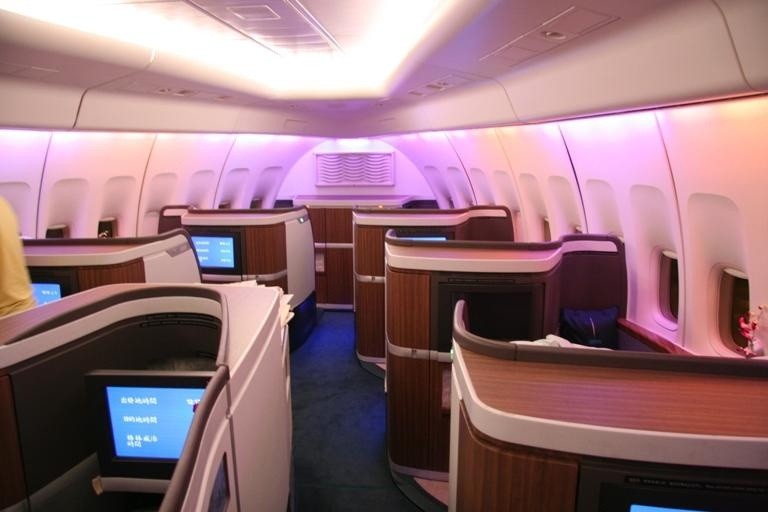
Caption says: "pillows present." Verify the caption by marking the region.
[560,304,621,351]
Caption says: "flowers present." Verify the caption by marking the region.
[735,306,765,359]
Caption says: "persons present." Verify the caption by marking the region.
[0,195,40,321]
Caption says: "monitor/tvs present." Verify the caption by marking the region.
[395,231,455,241]
[29,275,71,306]
[189,232,242,274]
[575,464,768,512]
[428,274,544,352]
[85,368,218,494]
